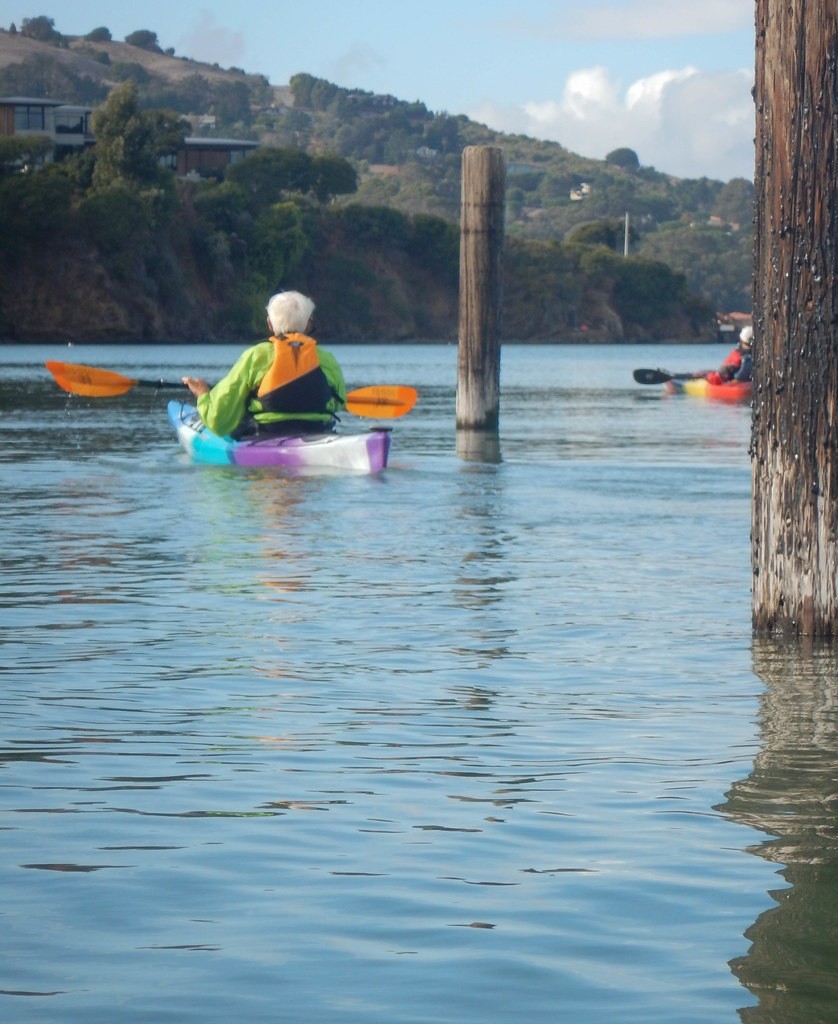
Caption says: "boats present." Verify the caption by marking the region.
[663,377,754,403]
[166,399,392,475]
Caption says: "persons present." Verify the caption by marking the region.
[706,325,753,386]
[182,290,347,442]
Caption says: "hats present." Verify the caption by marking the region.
[740,326,753,343]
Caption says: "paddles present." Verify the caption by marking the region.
[43,358,419,421]
[632,367,717,385]
